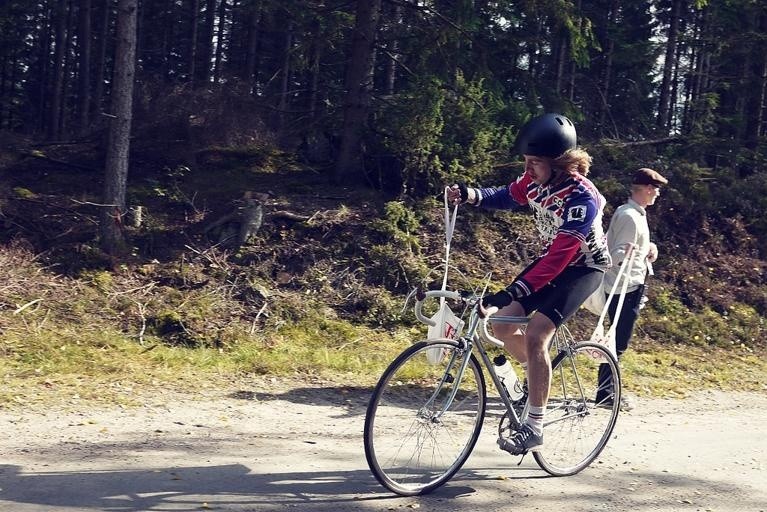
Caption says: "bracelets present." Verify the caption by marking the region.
[506,285,517,299]
[467,187,477,207]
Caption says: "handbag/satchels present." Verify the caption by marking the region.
[426,304,467,366]
[584,323,620,363]
[581,277,610,317]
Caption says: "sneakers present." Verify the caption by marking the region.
[497,424,544,453]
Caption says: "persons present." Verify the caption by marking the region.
[447,113,613,452]
[584,167,668,412]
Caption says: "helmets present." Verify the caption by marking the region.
[512,112,578,157]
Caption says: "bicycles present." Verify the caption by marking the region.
[364,262,623,496]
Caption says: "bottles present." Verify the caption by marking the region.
[494,355,524,401]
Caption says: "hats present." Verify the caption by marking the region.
[631,168,668,186]
